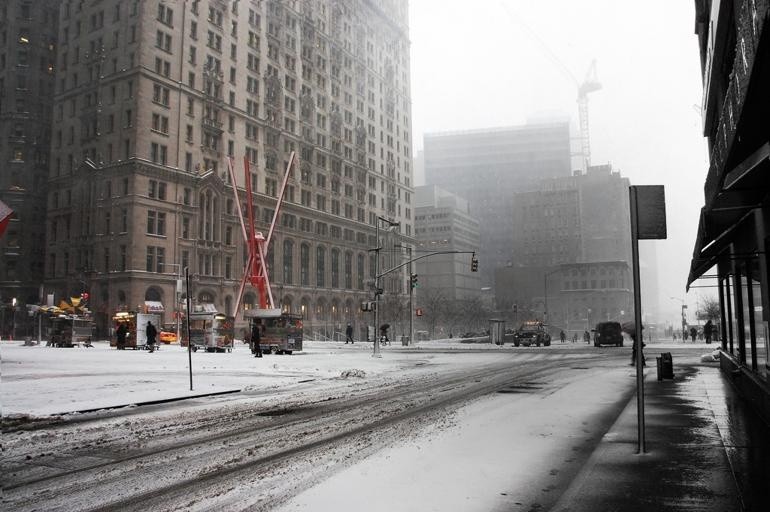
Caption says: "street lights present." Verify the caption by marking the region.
[369,216,399,358]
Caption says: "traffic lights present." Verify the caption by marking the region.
[411,272,418,288]
[471,258,478,271]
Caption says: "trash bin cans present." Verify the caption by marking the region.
[656,352,673,381]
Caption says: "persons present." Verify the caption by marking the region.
[690,327,697,342]
[115,320,129,349]
[628,332,646,367]
[250,323,263,358]
[380,329,390,342]
[344,323,354,344]
[145,320,158,353]
[559,330,566,342]
[703,319,712,343]
[585,330,591,344]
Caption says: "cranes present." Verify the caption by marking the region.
[570,58,603,171]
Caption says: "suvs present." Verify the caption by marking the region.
[513,319,550,346]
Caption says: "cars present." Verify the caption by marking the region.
[591,321,623,347]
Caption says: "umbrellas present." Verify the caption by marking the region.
[380,324,390,330]
[621,320,643,334]
